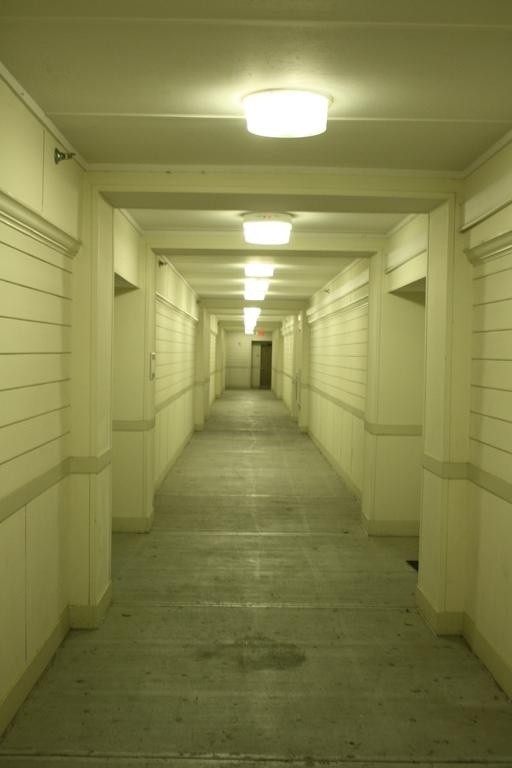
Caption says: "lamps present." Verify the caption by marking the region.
[242,211,294,246]
[243,307,262,335]
[243,281,270,293]
[241,87,331,139]
[243,262,275,278]
[243,293,266,301]
